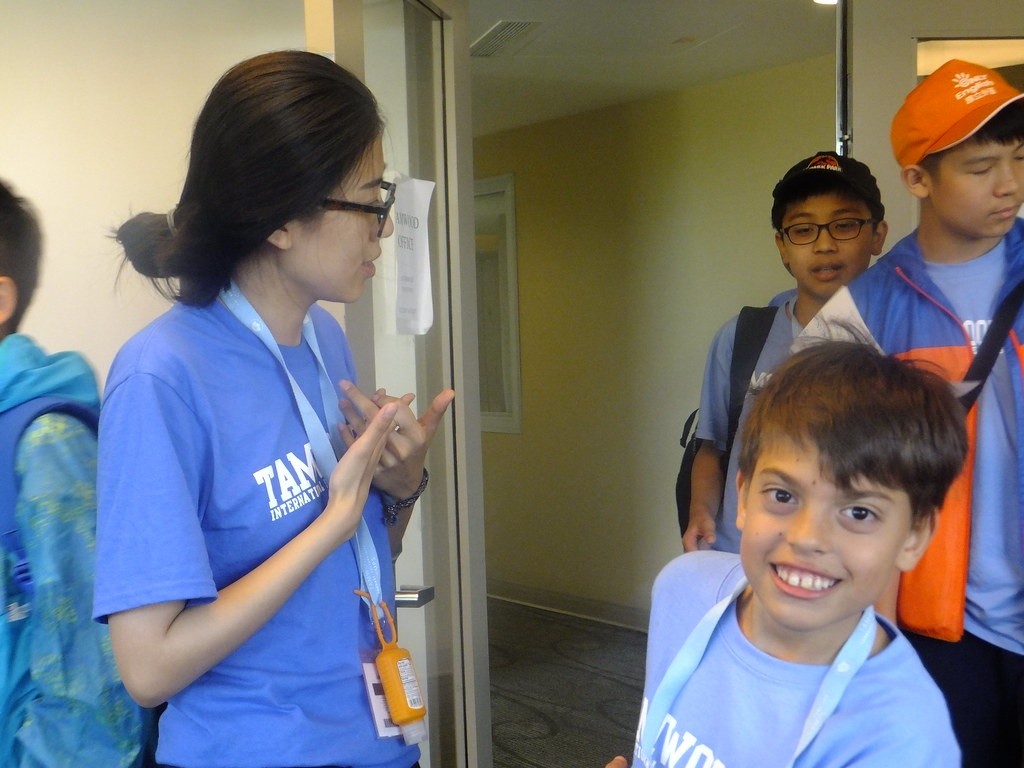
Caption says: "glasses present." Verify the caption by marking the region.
[316,182,398,237]
[780,218,872,245]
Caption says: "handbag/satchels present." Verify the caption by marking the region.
[676,306,778,537]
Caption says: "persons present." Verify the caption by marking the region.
[606,315,968,768]
[680,54,1024,768]
[0,173,157,768]
[93,50,456,768]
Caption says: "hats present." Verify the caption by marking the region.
[773,151,885,218]
[891,60,1024,168]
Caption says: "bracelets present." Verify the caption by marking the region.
[379,466,429,527]
